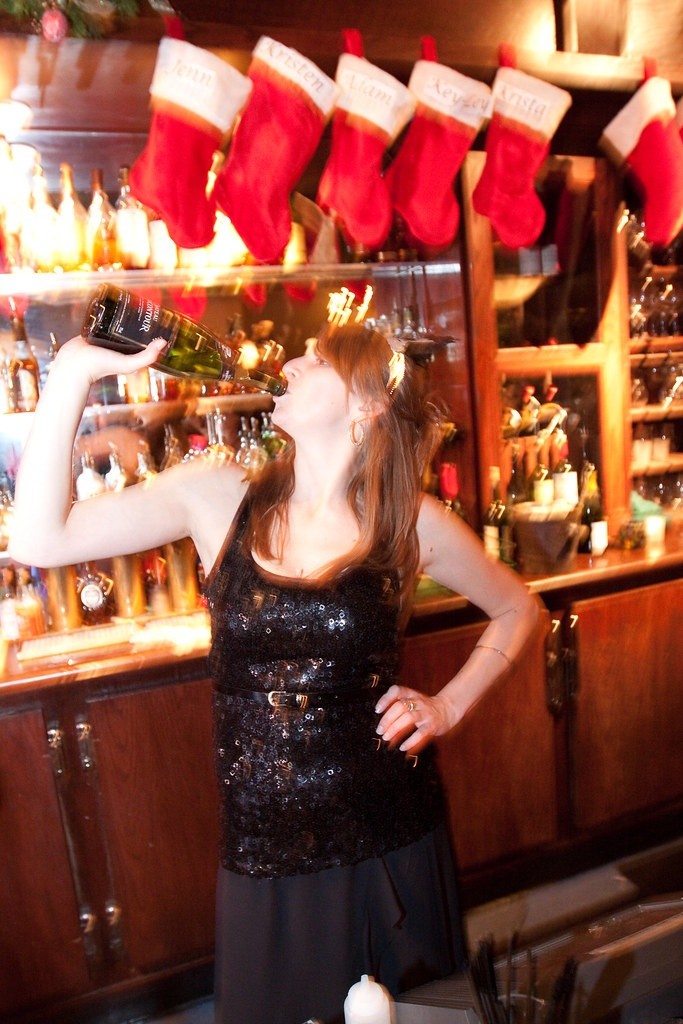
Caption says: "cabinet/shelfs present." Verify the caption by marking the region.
[3,119,682,674]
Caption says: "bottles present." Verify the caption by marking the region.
[0,161,683,643]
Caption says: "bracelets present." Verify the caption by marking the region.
[474,645,511,668]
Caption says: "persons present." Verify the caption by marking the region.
[8,320,541,1024]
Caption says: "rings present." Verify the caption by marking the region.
[400,697,415,712]
